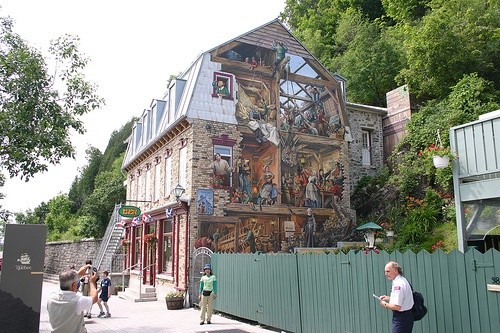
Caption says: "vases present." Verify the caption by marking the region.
[374,238,384,243]
[385,230,394,237]
[431,156,450,168]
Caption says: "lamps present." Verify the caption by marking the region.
[173,184,188,204]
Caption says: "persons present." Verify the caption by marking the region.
[197,263,218,325]
[379,261,414,333]
[46,259,112,333]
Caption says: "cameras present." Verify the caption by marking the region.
[88,265,93,274]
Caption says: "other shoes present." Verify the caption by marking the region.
[207,321,210,324]
[84,312,88,317]
[97,312,105,317]
[87,313,91,318]
[200,322,204,325]
[104,313,111,318]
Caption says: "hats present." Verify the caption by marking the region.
[203,264,211,270]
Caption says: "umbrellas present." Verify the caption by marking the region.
[356,221,382,230]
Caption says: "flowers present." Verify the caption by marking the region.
[142,234,157,249]
[419,143,455,164]
[381,223,394,231]
[374,232,386,238]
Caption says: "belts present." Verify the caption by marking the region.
[84,283,88,284]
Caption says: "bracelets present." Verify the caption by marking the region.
[384,303,388,308]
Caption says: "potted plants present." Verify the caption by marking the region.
[113,285,127,295]
[165,291,184,310]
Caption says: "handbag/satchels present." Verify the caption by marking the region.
[412,292,427,321]
[202,290,212,296]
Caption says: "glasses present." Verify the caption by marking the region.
[70,267,74,269]
